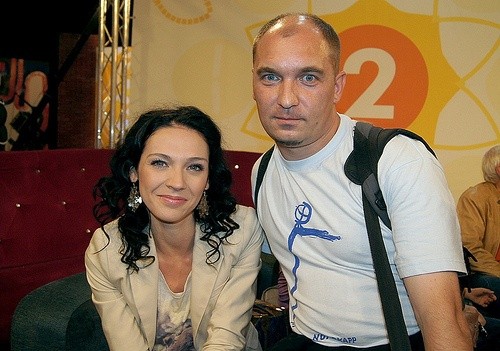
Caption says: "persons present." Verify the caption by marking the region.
[245,10,476,351]
[81,103,268,351]
[450,273,496,351]
[454,135,500,317]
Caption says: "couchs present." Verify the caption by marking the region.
[0,148,264,351]
